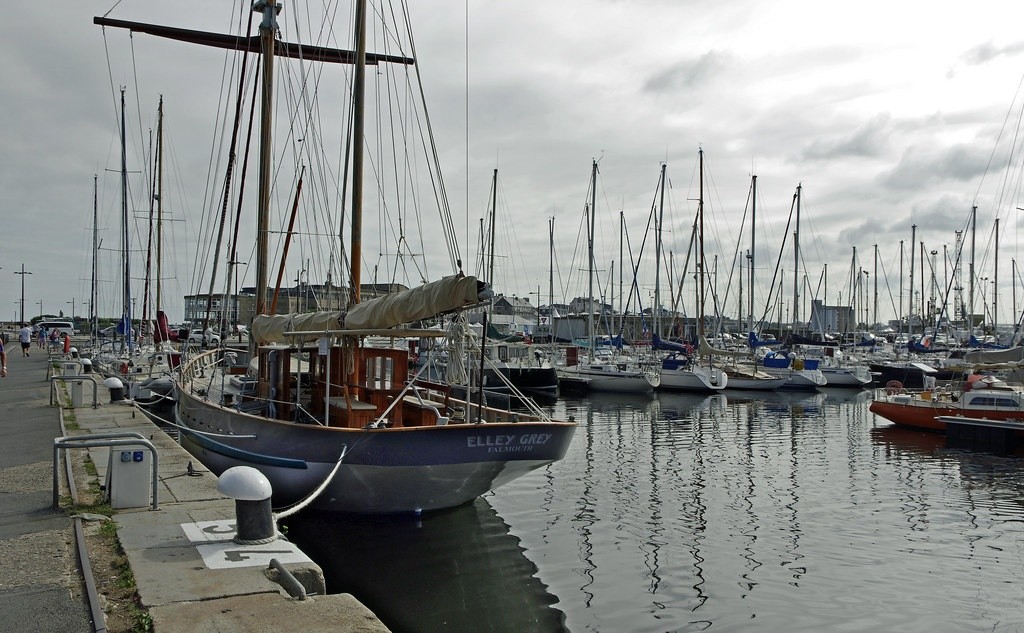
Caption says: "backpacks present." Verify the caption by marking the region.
[39,332,45,338]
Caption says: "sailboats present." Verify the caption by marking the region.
[537,147,1024,393]
[92,0,579,523]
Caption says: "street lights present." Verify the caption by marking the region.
[66,297,74,318]
[36,299,42,315]
[14,263,33,329]
[83,298,90,319]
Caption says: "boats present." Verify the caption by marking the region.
[869,374,1024,434]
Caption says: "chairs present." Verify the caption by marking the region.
[887,381,902,394]
[921,391,931,402]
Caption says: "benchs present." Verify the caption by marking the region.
[403,380,448,427]
[317,381,376,429]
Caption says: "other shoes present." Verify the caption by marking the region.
[26,352,29,357]
[37,346,39,349]
[22,353,25,357]
[43,347,45,349]
[1,368,8,377]
[40,346,42,349]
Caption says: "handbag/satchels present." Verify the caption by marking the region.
[50,335,53,340]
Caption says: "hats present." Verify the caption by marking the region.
[40,326,46,330]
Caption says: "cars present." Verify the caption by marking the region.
[100,325,135,337]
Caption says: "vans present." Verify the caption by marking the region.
[33,322,75,338]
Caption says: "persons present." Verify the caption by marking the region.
[0,338,7,377]
[523,332,533,345]
[19,322,61,357]
[201,336,207,347]
[220,327,228,346]
[318,333,332,374]
